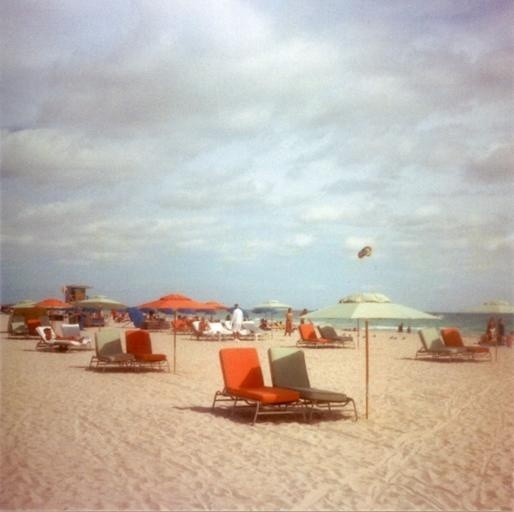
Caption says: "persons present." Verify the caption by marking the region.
[1,305,309,343]
[478,316,513,361]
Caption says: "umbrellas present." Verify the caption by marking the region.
[255,298,286,339]
[7,295,124,332]
[457,296,513,352]
[202,300,225,318]
[299,289,441,421]
[139,294,216,374]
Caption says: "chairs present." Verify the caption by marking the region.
[195,319,493,426]
[10,314,171,375]
